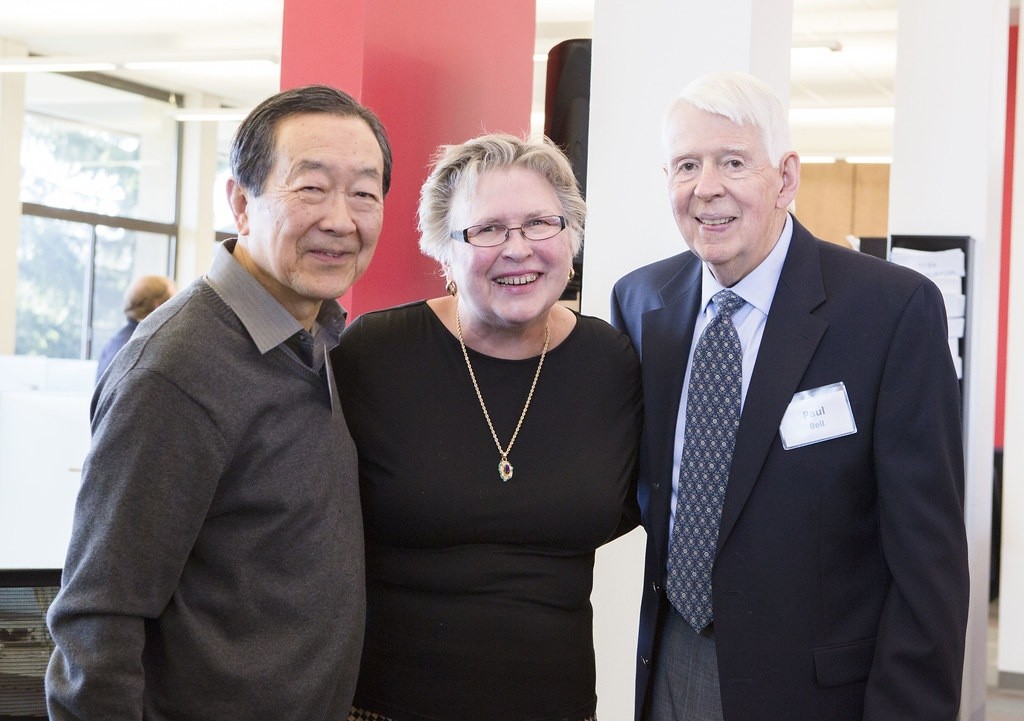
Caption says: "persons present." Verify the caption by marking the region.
[321,135,646,721]
[609,74,970,721]
[455,301,551,482]
[43,87,392,721]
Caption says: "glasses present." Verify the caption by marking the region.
[450,215,569,247]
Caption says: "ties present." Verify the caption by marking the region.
[666,290,746,634]
